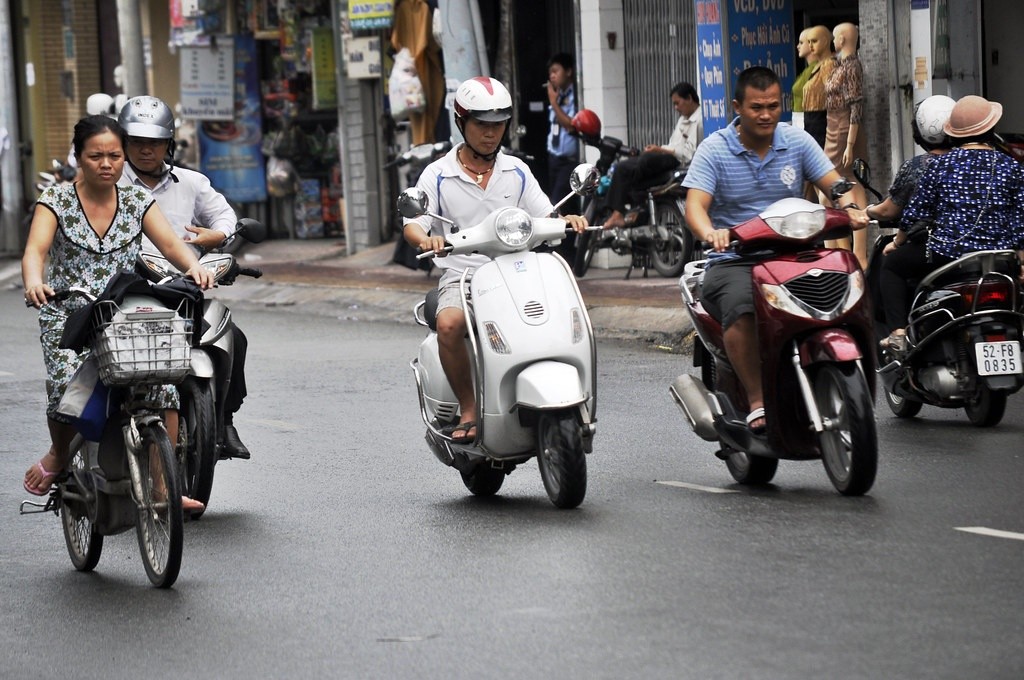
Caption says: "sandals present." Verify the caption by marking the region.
[153,497,205,516]
[452,419,477,442]
[879,333,907,356]
[22,461,60,496]
[746,407,768,432]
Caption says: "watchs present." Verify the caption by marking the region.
[843,203,860,209]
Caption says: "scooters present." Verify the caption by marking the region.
[852,158,1024,429]
[19,272,219,589]
[136,217,266,520]
[397,163,605,510]
[667,177,880,495]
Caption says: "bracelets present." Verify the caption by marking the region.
[866,203,874,218]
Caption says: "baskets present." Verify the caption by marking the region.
[85,298,194,387]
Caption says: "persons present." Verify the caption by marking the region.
[545,52,580,266]
[403,77,589,443]
[22,113,205,513]
[602,81,702,231]
[866,94,1024,358]
[802,26,837,203]
[813,23,863,251]
[792,28,818,131]
[68,93,117,170]
[680,67,871,434]
[119,95,250,459]
[114,65,122,86]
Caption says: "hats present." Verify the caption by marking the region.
[943,95,1002,138]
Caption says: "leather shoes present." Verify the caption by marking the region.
[221,425,250,459]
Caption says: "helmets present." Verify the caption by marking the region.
[915,95,957,144]
[454,77,513,130]
[118,96,175,139]
[570,110,601,141]
[85,93,114,116]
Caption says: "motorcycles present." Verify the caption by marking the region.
[566,129,694,279]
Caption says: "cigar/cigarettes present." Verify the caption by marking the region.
[542,84,548,86]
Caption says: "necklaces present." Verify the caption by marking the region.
[458,145,496,183]
